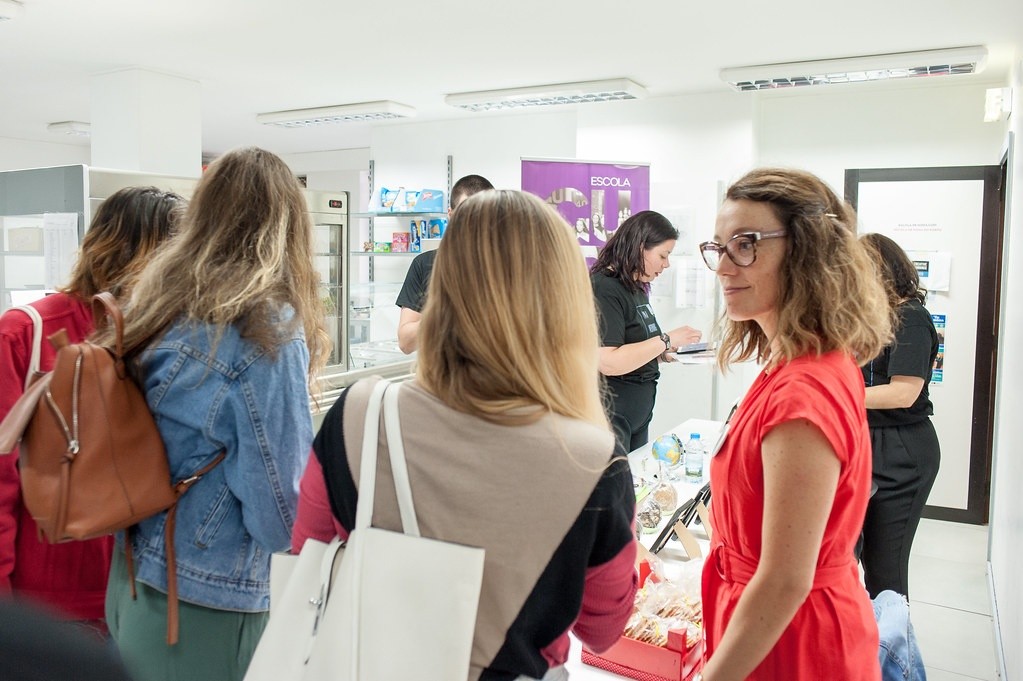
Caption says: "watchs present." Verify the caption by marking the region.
[660,333,671,350]
[692,673,702,681]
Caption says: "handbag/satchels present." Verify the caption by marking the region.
[240,377,486,681]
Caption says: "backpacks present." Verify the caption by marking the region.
[0,292,225,547]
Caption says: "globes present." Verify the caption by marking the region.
[651,433,684,470]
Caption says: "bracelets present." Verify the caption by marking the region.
[661,355,667,362]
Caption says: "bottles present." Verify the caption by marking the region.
[684,433,703,488]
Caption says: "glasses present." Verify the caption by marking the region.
[699,231,790,272]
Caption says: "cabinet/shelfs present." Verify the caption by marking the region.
[349,209,448,368]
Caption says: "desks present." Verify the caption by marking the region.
[515,415,725,681]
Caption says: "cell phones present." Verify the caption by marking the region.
[676,341,717,354]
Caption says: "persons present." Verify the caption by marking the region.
[104,145,334,681]
[587,210,701,453]
[851,233,940,602]
[690,168,897,681]
[575,218,589,236]
[592,214,603,233]
[618,207,632,218]
[395,174,496,355]
[0,185,190,636]
[291,189,640,681]
